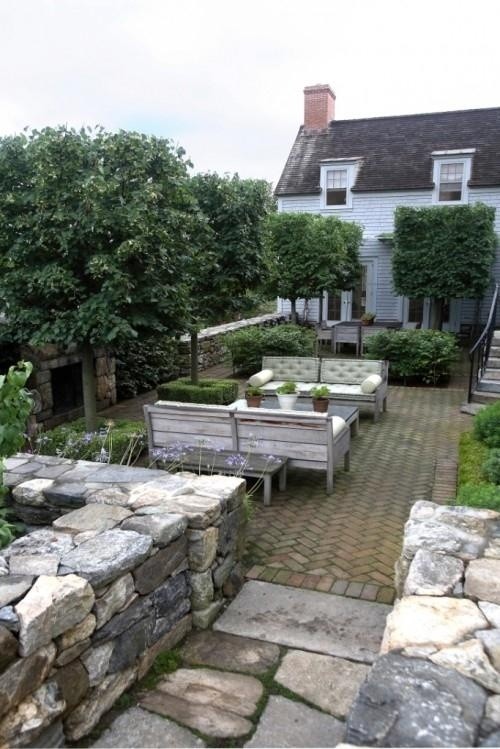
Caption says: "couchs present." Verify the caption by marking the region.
[245,360,389,422]
[142,403,351,495]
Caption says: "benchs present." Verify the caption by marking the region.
[150,444,290,506]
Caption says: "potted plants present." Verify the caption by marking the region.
[360,312,376,326]
[361,328,462,388]
[244,384,266,408]
[309,386,330,412]
[274,382,301,409]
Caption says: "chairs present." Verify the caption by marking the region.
[314,318,389,359]
